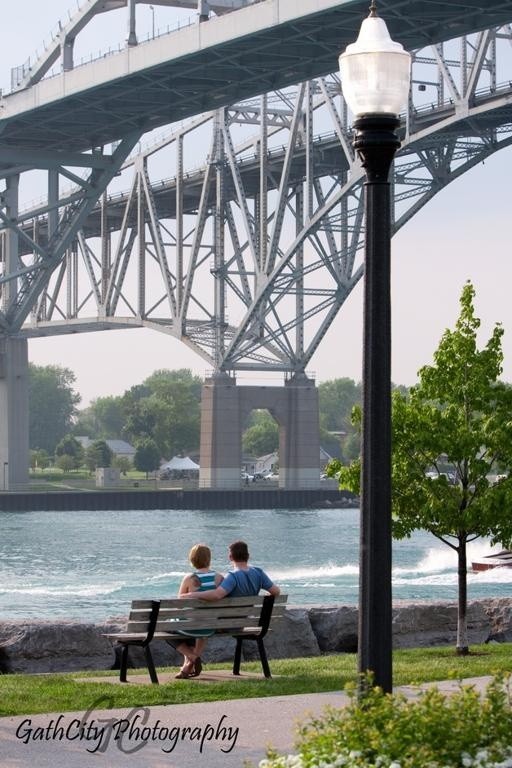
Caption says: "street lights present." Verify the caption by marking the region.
[337,0,411,690]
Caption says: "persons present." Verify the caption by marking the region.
[164,544,225,679]
[179,540,281,678]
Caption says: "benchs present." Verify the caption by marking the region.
[101,593,289,686]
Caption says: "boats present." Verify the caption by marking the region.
[473,550,512,572]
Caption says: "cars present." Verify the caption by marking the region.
[241,468,279,483]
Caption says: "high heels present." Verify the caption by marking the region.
[174,656,206,678]
